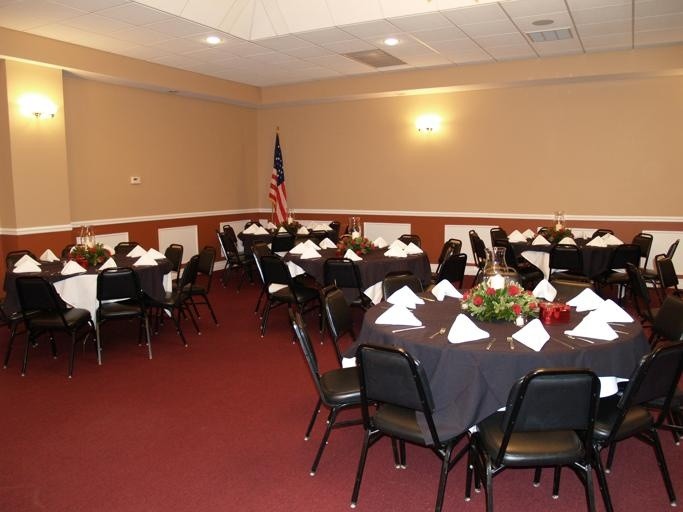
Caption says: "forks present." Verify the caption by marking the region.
[567,336,594,344]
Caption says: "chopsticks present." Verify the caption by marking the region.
[392,326,426,334]
[421,298,434,302]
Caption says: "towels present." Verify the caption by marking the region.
[387,286,425,309]
[387,239,408,249]
[567,287,607,311]
[586,236,608,247]
[40,248,60,262]
[296,226,309,235]
[242,223,259,234]
[600,233,624,245]
[307,223,333,231]
[507,229,527,242]
[288,242,307,255]
[299,244,322,259]
[60,260,87,275]
[405,242,423,254]
[522,228,536,238]
[564,312,619,341]
[13,254,42,267]
[303,239,321,250]
[277,227,287,233]
[126,244,147,257]
[98,257,117,270]
[447,313,490,344]
[133,253,158,266]
[511,318,550,352]
[383,245,407,258]
[265,222,278,229]
[432,279,465,302]
[531,235,550,245]
[488,273,506,290]
[532,279,558,301]
[13,259,41,273]
[370,236,390,248]
[252,226,269,235]
[318,237,337,249]
[343,249,363,262]
[376,302,423,326]
[594,299,636,323]
[146,248,166,260]
[557,236,577,245]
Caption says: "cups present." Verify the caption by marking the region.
[80,225,94,250]
[552,209,565,231]
[483,247,516,284]
[349,216,361,238]
[287,208,296,224]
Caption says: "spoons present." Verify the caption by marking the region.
[505,337,514,350]
[429,328,445,342]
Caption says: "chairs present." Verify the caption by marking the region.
[648,292,683,350]
[96,266,144,352]
[0,299,42,369]
[496,237,541,284]
[163,243,184,274]
[624,260,661,346]
[323,282,358,367]
[61,243,88,257]
[475,365,615,512]
[219,225,247,283]
[399,234,422,249]
[634,231,654,270]
[322,257,371,309]
[489,227,508,246]
[328,220,341,246]
[344,223,359,235]
[431,238,463,280]
[435,253,468,289]
[471,234,487,285]
[547,243,585,282]
[348,342,476,512]
[114,241,141,255]
[591,342,682,512]
[381,275,424,301]
[641,238,681,291]
[5,248,39,270]
[271,231,295,254]
[287,306,406,477]
[13,272,100,379]
[655,255,683,301]
[244,221,263,231]
[592,243,643,307]
[183,244,220,327]
[138,253,202,347]
[257,255,324,345]
[307,230,329,246]
[215,229,256,294]
[593,227,615,238]
[468,229,481,278]
[250,243,273,321]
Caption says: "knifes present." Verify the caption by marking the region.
[551,336,574,351]
[485,337,496,350]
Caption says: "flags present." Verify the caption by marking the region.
[267,132,287,226]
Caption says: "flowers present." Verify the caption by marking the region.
[458,276,541,323]
[334,236,374,258]
[535,228,575,245]
[279,221,302,230]
[64,242,112,269]
